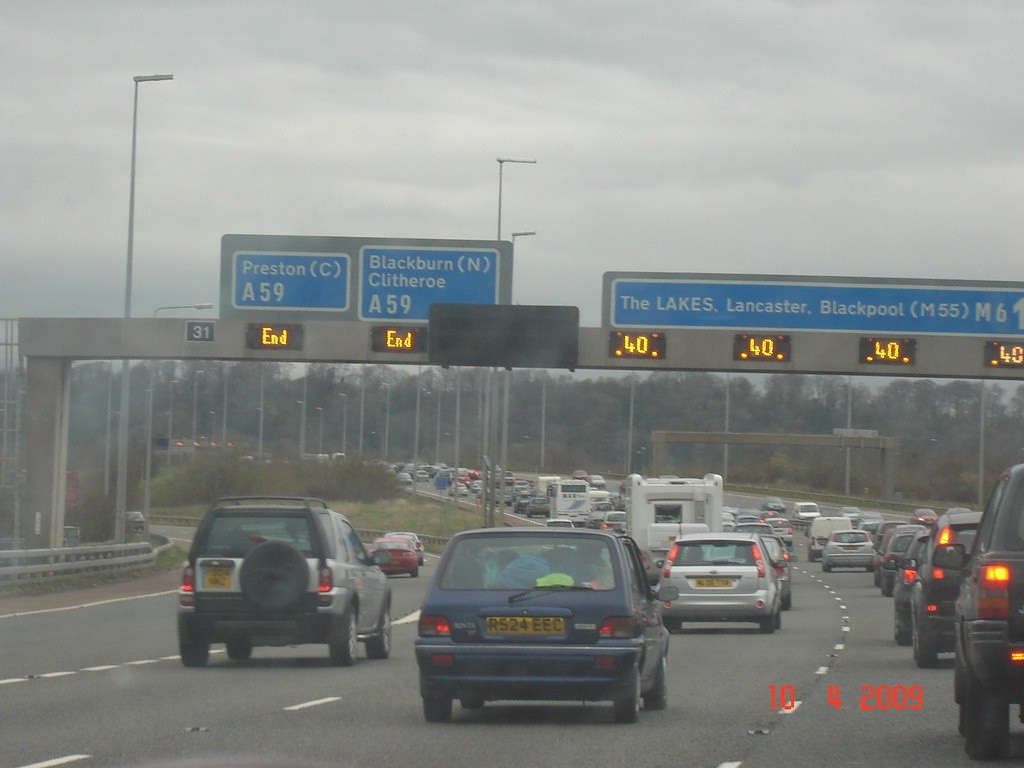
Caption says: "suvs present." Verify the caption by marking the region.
[176,495,395,669]
[931,461,1024,761]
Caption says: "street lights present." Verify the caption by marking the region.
[495,158,542,240]
[145,303,217,542]
[294,401,304,453]
[314,406,323,457]
[190,369,204,444]
[336,392,346,452]
[496,229,539,516]
[166,380,180,467]
[115,72,176,545]
[382,382,389,461]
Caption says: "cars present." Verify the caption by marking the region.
[417,526,682,722]
[390,457,886,612]
[882,526,931,646]
[655,533,786,637]
[910,507,939,523]
[899,510,984,670]
[365,530,423,578]
[943,507,972,515]
[126,511,145,530]
